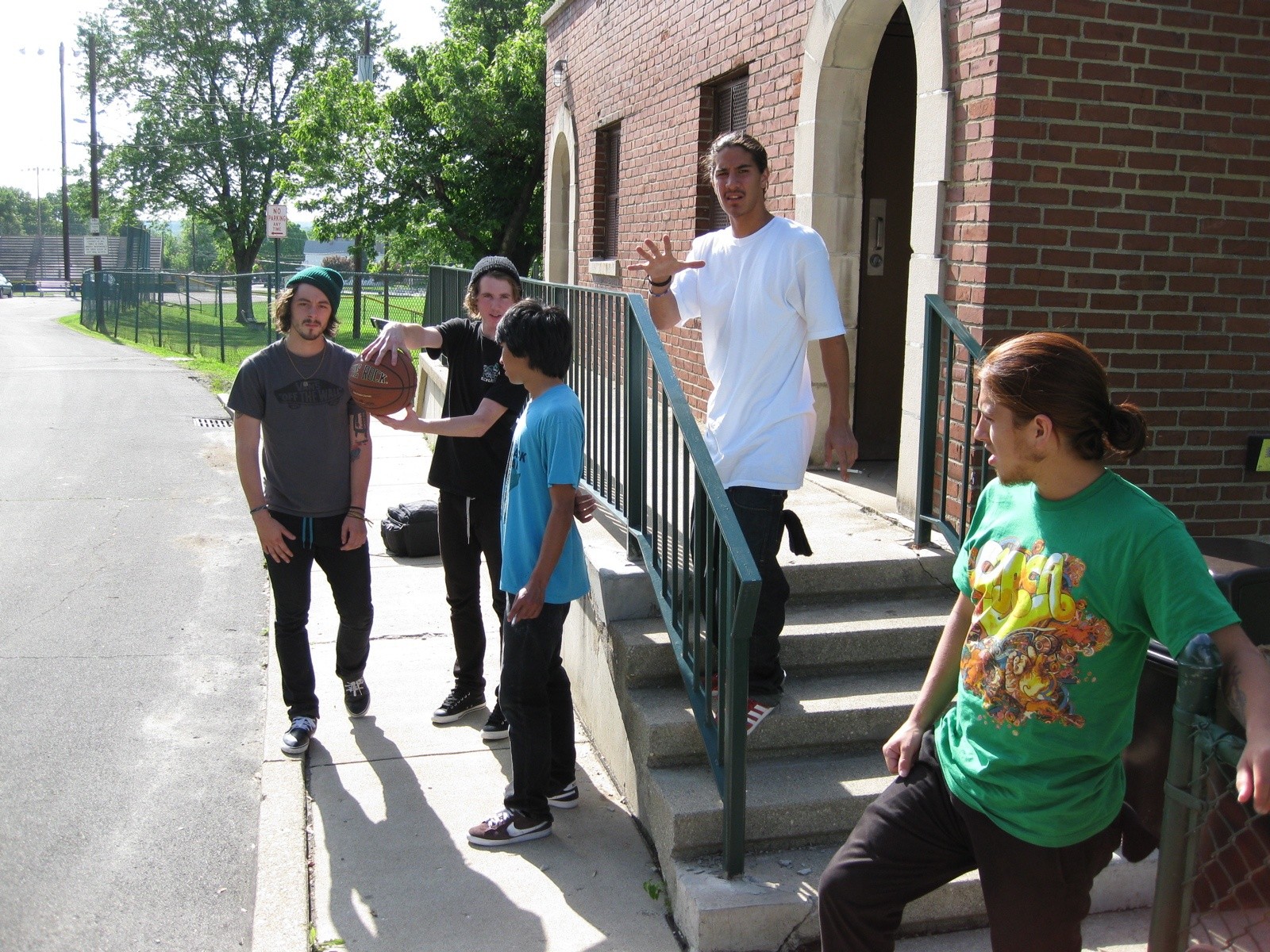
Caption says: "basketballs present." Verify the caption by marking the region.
[348,348,417,416]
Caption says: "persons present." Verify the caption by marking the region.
[228,267,374,754]
[361,257,527,740]
[628,130,859,741]
[817,333,1270,952]
[467,299,597,845]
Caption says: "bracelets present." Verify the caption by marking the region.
[648,289,671,297]
[250,505,268,514]
[347,506,374,527]
[647,274,671,286]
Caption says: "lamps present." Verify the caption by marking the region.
[553,60,566,87]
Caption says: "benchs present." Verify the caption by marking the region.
[36,281,71,298]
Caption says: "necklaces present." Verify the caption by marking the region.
[284,337,327,387]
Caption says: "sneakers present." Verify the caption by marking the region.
[280,717,317,754]
[481,704,509,739]
[431,689,486,722]
[466,811,552,846]
[342,675,371,718]
[715,698,778,735]
[546,781,580,809]
[701,667,786,696]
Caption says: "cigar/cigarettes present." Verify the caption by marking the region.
[837,467,862,474]
[511,614,517,627]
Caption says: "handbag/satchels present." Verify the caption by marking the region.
[381,499,441,557]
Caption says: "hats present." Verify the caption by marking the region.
[468,256,524,297]
[286,265,343,315]
